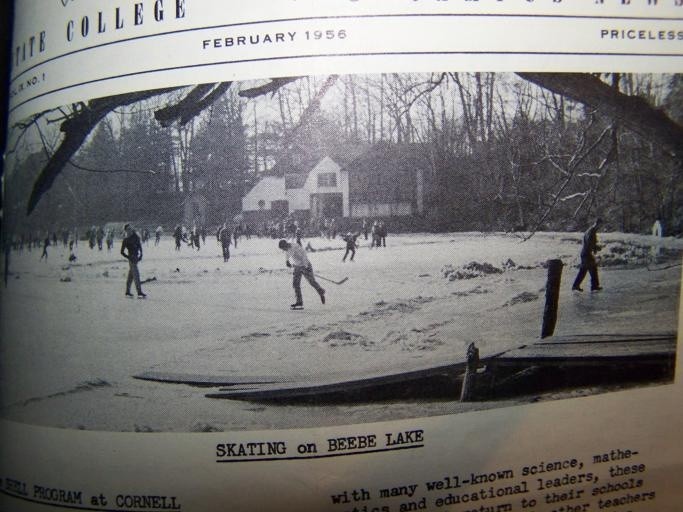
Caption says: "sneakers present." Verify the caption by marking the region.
[125,293,134,296]
[138,293,146,296]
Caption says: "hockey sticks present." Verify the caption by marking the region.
[290,265,349,286]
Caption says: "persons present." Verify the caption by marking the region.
[12,226,163,263]
[172,218,337,262]
[572,217,604,292]
[279,240,325,307]
[340,232,360,263]
[121,224,146,297]
[363,218,387,247]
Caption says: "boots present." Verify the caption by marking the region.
[320,289,325,304]
[291,301,303,307]
